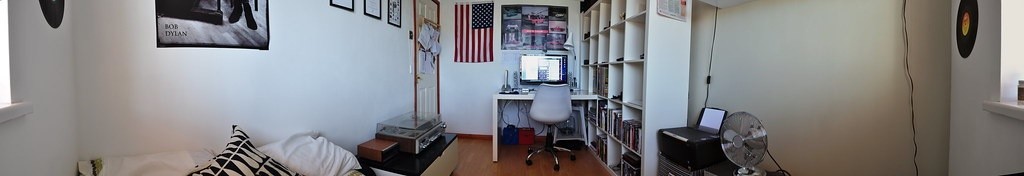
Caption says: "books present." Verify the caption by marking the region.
[587,101,597,124]
[622,119,643,153]
[592,67,598,94]
[622,151,641,176]
[597,67,608,97]
[609,108,623,140]
[589,134,608,165]
[598,99,608,131]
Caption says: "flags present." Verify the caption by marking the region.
[454,3,494,63]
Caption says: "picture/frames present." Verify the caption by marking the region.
[155,0,269,50]
[388,0,401,28]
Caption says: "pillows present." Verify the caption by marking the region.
[77,145,217,176]
[255,126,362,176]
[185,123,305,176]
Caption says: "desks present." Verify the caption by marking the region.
[493,89,597,162]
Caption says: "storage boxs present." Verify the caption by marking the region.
[503,128,518,144]
[519,128,535,145]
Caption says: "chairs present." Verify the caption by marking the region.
[525,83,577,171]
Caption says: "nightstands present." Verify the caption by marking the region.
[355,133,459,176]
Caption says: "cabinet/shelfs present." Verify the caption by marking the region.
[580,0,692,176]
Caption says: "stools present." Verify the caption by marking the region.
[552,104,588,145]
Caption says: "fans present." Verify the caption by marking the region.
[719,111,768,176]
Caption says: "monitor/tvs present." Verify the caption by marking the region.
[519,54,567,85]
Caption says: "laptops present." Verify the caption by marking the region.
[666,107,728,140]
